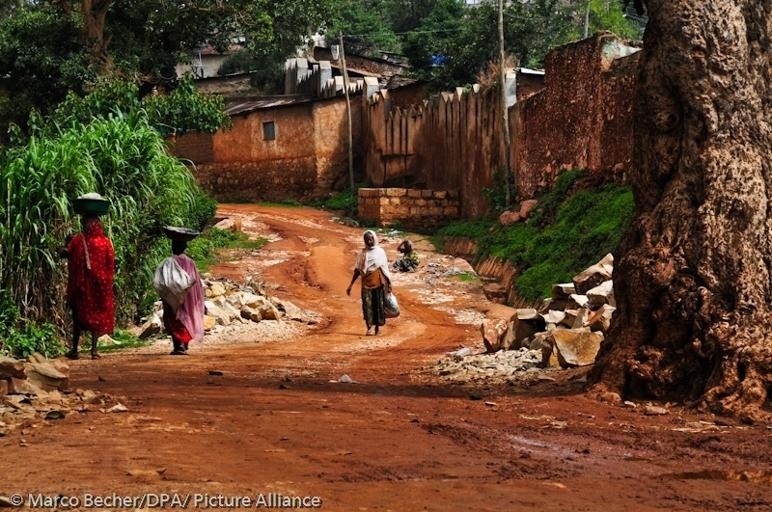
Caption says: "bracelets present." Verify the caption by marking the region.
[63,246,69,250]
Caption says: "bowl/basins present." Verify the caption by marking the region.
[72,199,110,215]
[164,225,199,241]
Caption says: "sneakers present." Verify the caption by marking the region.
[62,350,103,361]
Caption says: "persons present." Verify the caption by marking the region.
[153,238,203,356]
[346,229,393,336]
[391,240,419,273]
[60,213,116,359]
